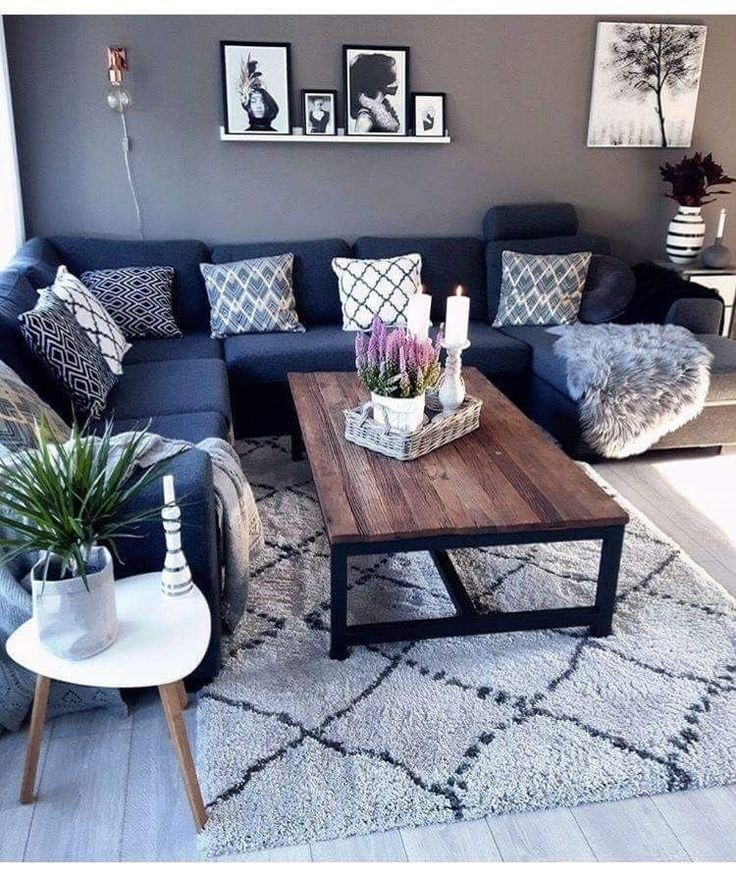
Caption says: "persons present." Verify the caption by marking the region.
[241,88,279,132]
[310,96,330,132]
[350,54,400,133]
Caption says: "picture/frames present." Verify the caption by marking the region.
[300,89,338,136]
[410,92,447,137]
[341,44,410,136]
[219,40,291,136]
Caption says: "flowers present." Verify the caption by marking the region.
[658,149,735,206]
[356,314,442,392]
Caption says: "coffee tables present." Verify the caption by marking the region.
[285,366,631,665]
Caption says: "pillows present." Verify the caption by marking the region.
[579,254,637,323]
[0,358,71,449]
[52,261,133,376]
[330,252,434,332]
[79,266,183,338]
[493,249,592,328]
[17,287,119,419]
[199,252,305,339]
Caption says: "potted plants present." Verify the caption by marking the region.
[6,414,156,663]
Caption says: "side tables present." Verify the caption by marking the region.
[6,572,213,836]
[654,258,736,337]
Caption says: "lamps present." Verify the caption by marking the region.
[101,45,131,114]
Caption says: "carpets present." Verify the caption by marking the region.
[194,428,736,861]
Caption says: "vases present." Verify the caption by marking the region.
[369,388,426,430]
[666,204,705,264]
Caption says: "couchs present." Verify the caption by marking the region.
[0,203,736,734]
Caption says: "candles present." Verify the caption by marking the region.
[163,472,177,508]
[716,208,726,237]
[407,283,432,338]
[445,284,471,343]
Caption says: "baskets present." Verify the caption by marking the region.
[344,371,484,461]
